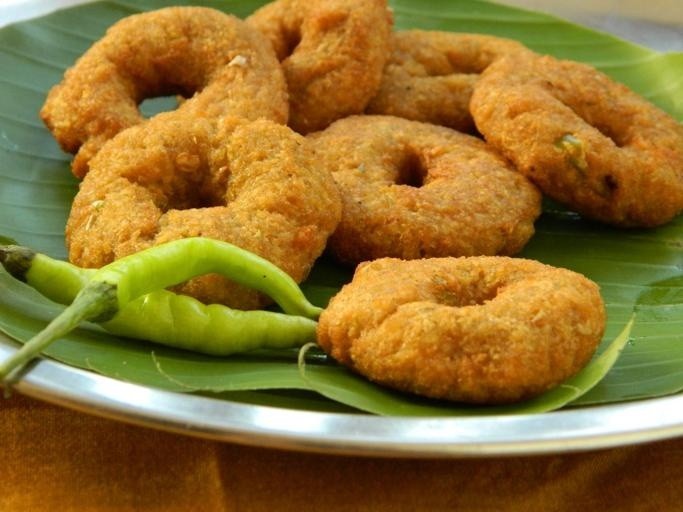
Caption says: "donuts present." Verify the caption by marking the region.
[65,109,344,310]
[315,255,606,405]
[368,30,530,117]
[313,113,543,275]
[468,50,683,230]
[39,7,290,180]
[242,0,392,125]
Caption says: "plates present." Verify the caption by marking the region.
[0,0,683,459]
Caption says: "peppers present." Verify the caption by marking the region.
[1,243,317,352]
[1,236,324,378]
